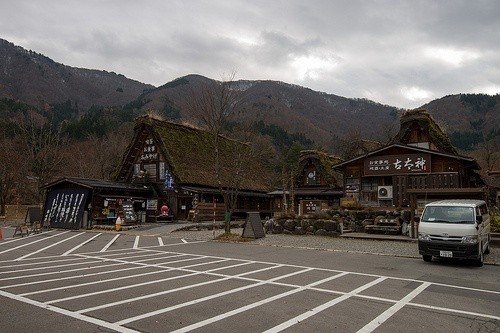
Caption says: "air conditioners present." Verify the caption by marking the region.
[378,186,392,198]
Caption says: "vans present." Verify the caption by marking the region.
[417,198,491,267]
